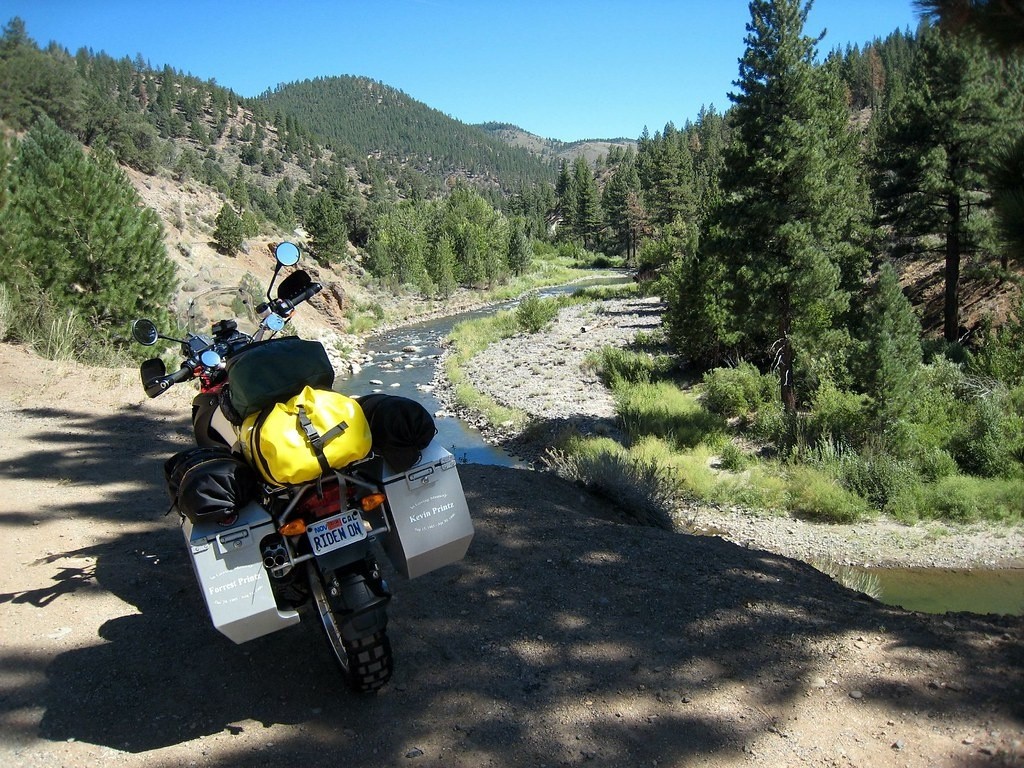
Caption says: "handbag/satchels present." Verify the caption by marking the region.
[230,384,373,494]
[162,446,258,526]
[355,393,438,474]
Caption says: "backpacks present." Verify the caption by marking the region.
[218,335,335,426]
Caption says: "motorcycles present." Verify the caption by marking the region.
[129,242,474,695]
